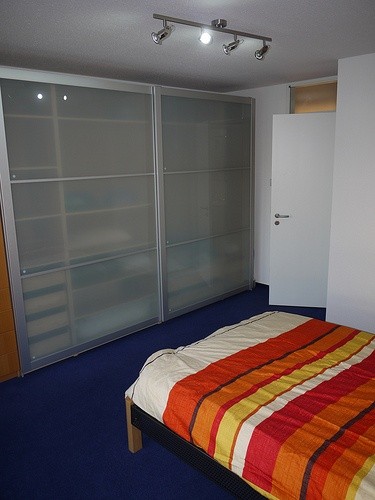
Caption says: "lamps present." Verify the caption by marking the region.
[254,39,270,60]
[222,34,243,54]
[152,18,175,44]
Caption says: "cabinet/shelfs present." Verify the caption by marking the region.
[0,65,256,377]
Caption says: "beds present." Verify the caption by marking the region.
[126,310,375,500]
